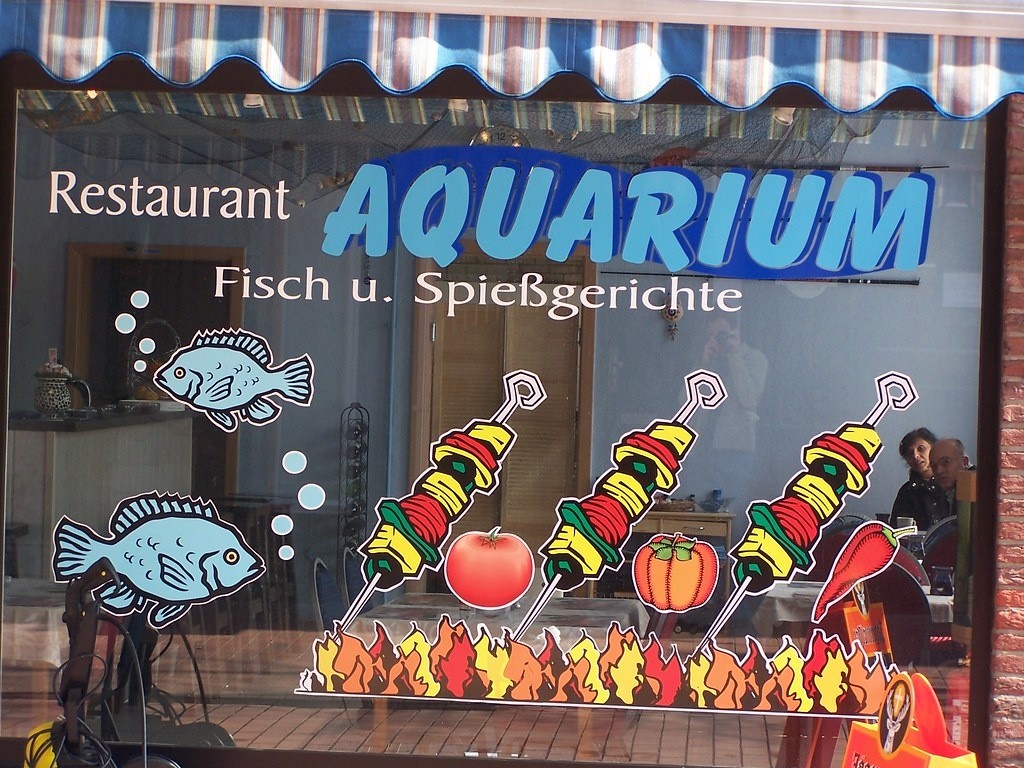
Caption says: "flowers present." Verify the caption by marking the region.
[38,362,69,378]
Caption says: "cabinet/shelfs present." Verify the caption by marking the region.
[8,400,193,656]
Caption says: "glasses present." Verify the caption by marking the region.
[927,456,964,471]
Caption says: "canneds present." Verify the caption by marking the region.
[712,488,722,500]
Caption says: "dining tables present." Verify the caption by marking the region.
[894,528,928,559]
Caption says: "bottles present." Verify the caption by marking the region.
[342,518,364,537]
[346,480,364,498]
[690,488,733,512]
[345,441,366,459]
[344,537,363,555]
[344,499,365,517]
[346,422,367,440]
[346,460,364,479]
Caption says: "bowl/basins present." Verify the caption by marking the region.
[66,399,160,419]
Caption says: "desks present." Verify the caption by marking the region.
[750,582,955,650]
[585,509,736,633]
[346,592,650,643]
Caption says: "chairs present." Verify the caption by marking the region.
[809,514,866,581]
[311,557,350,637]
[342,546,373,612]
[159,497,298,672]
[921,515,960,579]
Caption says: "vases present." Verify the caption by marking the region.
[35,372,72,421]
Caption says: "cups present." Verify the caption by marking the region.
[875,513,890,524]
[897,516,916,528]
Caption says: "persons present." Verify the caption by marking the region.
[929,437,978,521]
[674,305,773,513]
[890,425,955,534]
